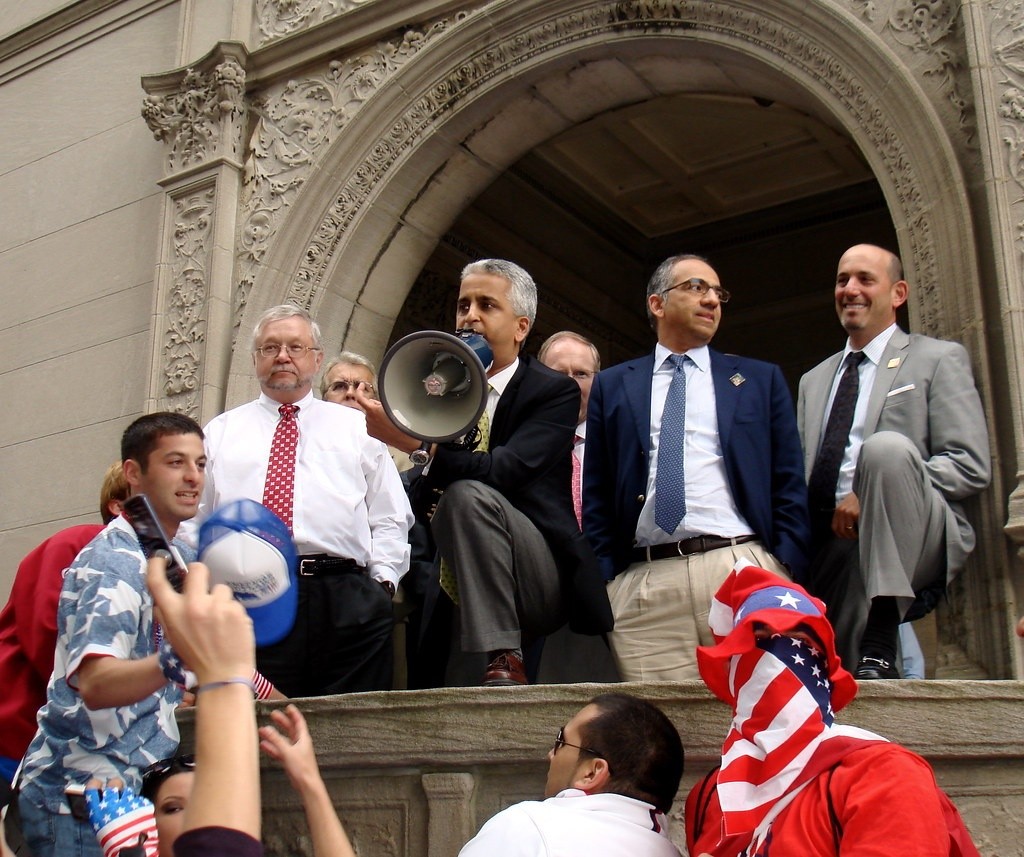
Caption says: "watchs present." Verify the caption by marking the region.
[384,581,395,598]
[408,442,432,465]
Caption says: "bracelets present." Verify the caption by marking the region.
[197,677,254,695]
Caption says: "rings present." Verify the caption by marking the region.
[846,527,854,529]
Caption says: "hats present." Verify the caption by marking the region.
[696,556,859,712]
[196,498,299,645]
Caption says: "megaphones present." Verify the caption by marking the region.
[377,329,494,445]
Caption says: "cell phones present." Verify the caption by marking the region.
[125,494,189,594]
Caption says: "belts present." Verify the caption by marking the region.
[294,555,363,576]
[630,535,752,563]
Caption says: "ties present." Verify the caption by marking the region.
[808,351,866,537]
[654,356,691,536]
[263,404,299,537]
[571,435,582,531]
[440,384,494,605]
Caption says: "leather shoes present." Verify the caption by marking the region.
[482,652,528,686]
[855,656,901,680]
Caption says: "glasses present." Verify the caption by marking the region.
[138,754,199,798]
[257,342,320,359]
[325,380,378,399]
[554,728,603,758]
[664,278,730,303]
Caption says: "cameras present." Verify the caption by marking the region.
[66,785,103,823]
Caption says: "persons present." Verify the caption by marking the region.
[686,556,951,857]
[458,693,685,857]
[797,243,991,680]
[7,412,287,857]
[355,258,583,685]
[84,558,355,857]
[319,350,463,689]
[172,305,417,698]
[534,331,622,684]
[0,459,131,816]
[580,254,809,681]
